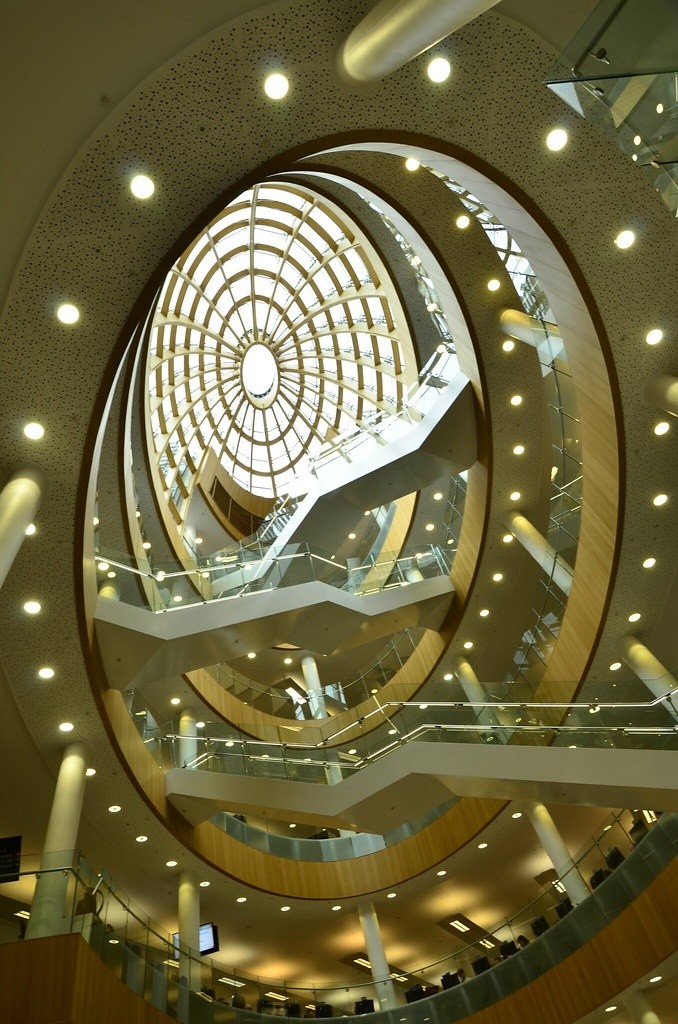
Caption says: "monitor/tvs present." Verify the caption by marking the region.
[172,922,216,960]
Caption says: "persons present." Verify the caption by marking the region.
[517,936,530,948]
[457,968,473,984]
